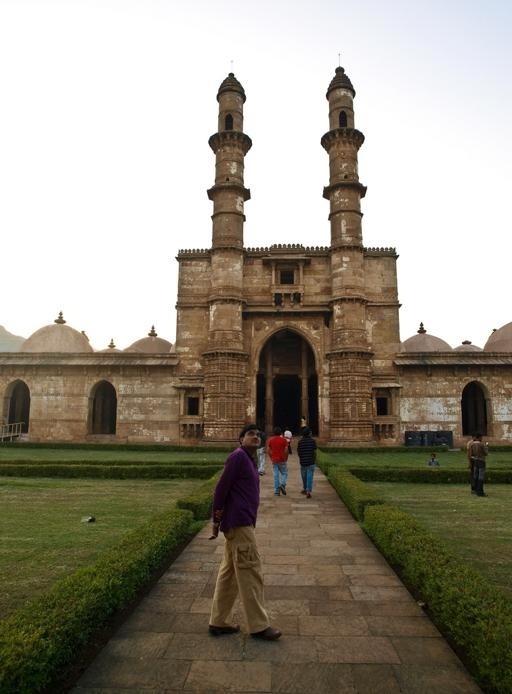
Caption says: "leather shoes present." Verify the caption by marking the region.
[307,491,311,498]
[250,626,282,641]
[302,491,306,494]
[209,624,240,636]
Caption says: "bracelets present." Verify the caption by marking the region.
[211,521,220,528]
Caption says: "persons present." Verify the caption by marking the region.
[208,424,282,640]
[467,432,476,490]
[300,416,307,438]
[255,427,267,475]
[284,427,292,454]
[266,427,289,497]
[470,431,488,497]
[297,427,317,498]
[428,453,440,466]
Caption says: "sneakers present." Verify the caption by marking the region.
[274,492,280,496]
[279,483,286,495]
